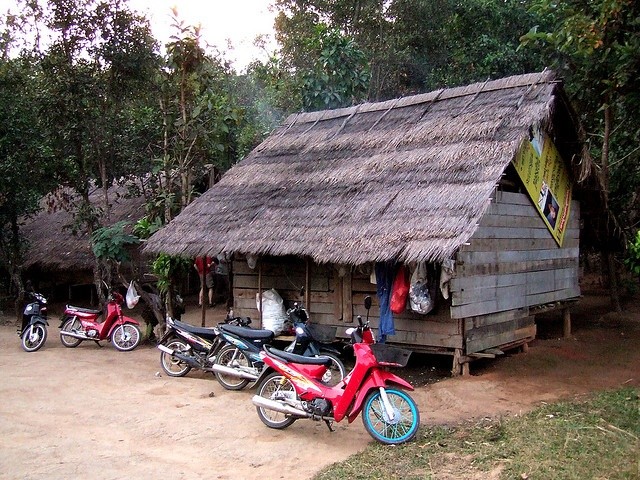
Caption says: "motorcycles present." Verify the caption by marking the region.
[16,276,51,353]
[254,295,419,443]
[212,286,347,389]
[156,307,252,376]
[58,276,141,351]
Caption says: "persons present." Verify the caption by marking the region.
[528,125,544,156]
[193,256,217,309]
[543,189,560,230]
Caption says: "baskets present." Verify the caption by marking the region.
[369,342,413,368]
[306,324,338,342]
